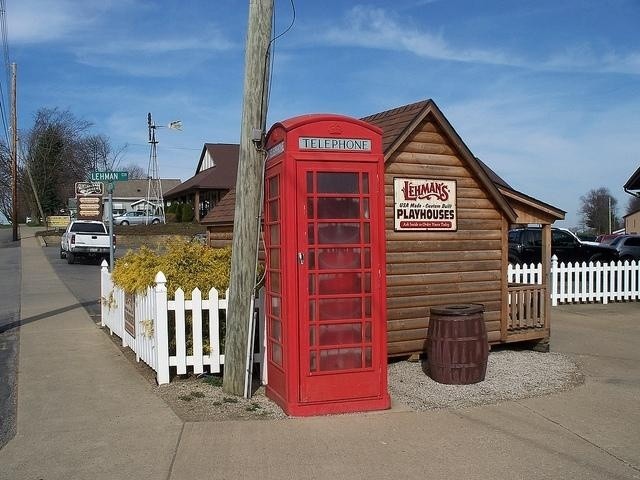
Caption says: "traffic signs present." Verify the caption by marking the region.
[90,171,129,182]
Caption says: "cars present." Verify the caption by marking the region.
[595,232,640,262]
[113,210,164,226]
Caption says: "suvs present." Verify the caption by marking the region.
[507,227,621,282]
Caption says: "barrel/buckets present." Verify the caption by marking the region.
[428,302,488,384]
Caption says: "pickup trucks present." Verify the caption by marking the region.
[59,219,117,265]
